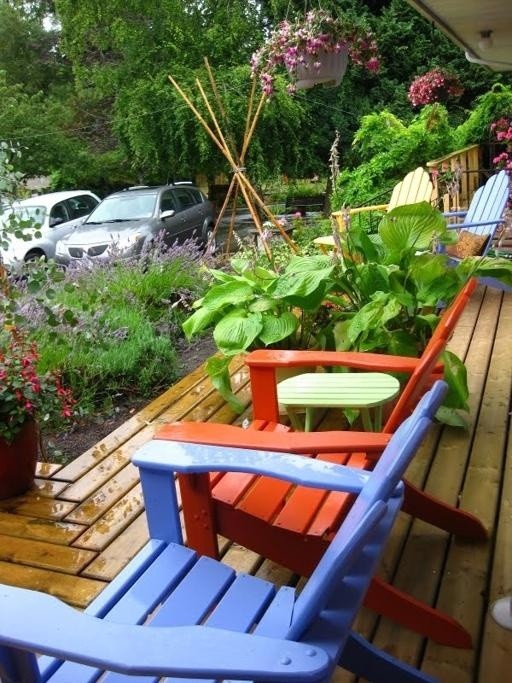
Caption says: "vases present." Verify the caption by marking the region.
[276,50,353,90]
[2,417,40,497]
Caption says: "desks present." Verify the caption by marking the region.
[275,371,395,429]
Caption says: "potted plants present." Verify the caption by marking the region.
[181,248,337,412]
[283,207,471,430]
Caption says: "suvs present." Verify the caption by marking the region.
[55,181,218,270]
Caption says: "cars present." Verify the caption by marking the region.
[0,188,103,279]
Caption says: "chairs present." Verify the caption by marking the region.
[310,164,510,309]
[170,278,486,649]
[1,378,452,683]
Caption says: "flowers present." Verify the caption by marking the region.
[407,65,464,107]
[248,6,380,96]
[1,278,78,432]
